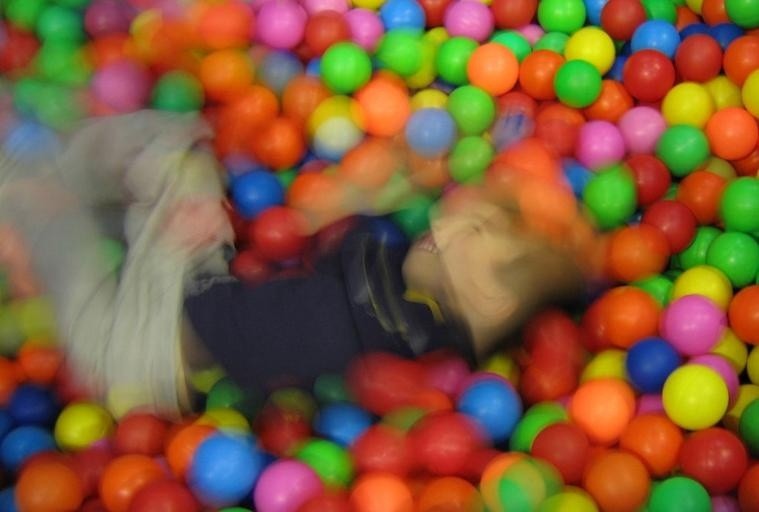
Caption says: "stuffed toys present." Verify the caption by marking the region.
[0,0,759,512]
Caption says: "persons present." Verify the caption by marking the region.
[0,86,600,429]
